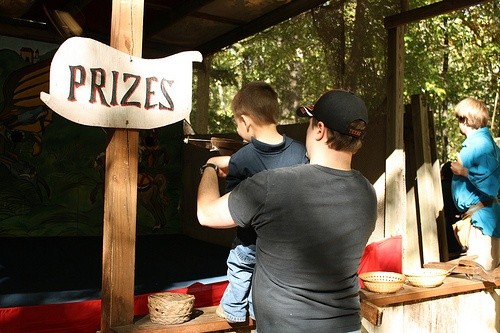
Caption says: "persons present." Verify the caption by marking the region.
[450,97,500,271]
[197,80,377,333]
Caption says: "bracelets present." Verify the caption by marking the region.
[200,163,219,177]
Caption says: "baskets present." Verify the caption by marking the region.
[148,293,195,326]
[404,268,448,288]
[358,271,406,293]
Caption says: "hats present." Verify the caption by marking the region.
[296,91,369,139]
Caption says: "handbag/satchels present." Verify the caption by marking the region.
[452,215,471,251]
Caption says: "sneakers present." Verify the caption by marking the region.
[215,305,240,324]
